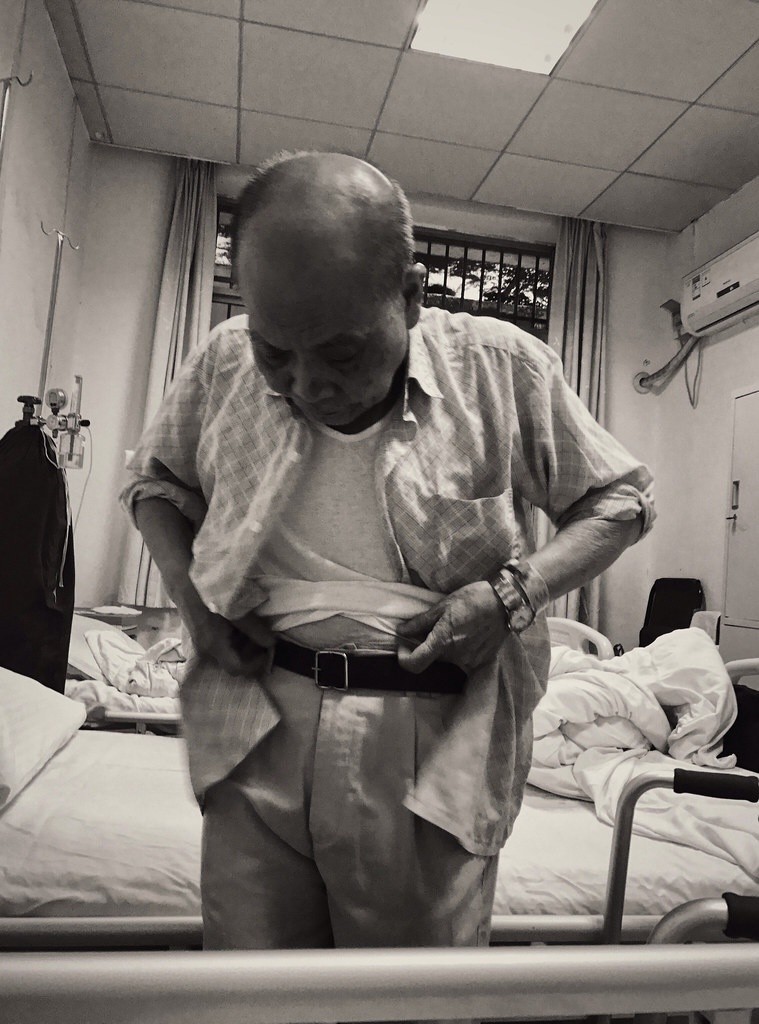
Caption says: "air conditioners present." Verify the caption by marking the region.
[677,230,759,340]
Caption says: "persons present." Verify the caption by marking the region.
[114,146,657,949]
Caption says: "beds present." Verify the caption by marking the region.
[1,656,759,1023]
[66,610,614,737]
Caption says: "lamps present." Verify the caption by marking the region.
[409,0,599,76]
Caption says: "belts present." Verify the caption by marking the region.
[276,635,463,697]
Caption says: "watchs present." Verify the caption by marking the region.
[485,569,538,635]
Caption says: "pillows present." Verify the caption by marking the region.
[0,664,90,810]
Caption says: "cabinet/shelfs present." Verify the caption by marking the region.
[716,378,758,689]
